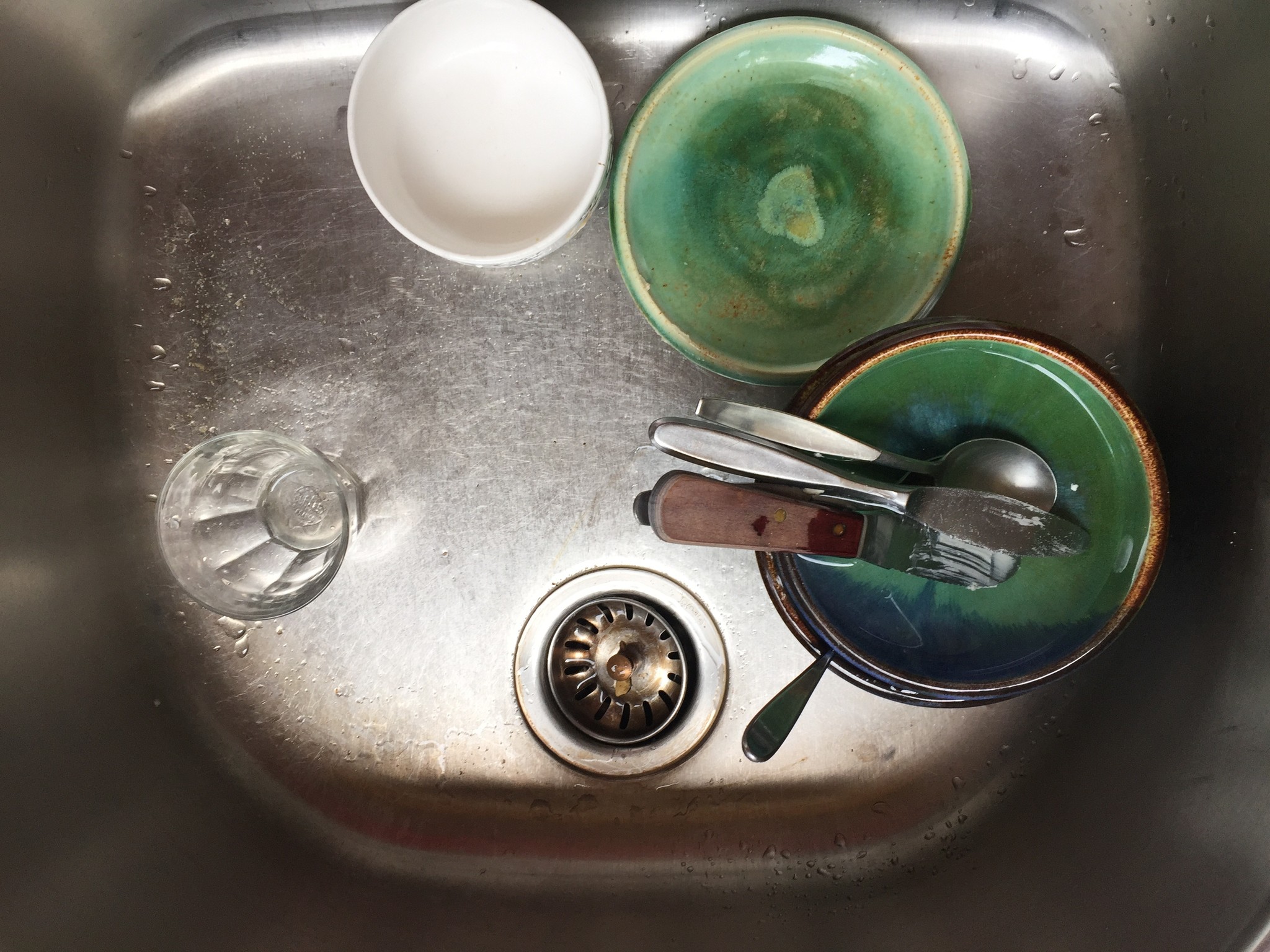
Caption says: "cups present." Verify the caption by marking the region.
[156,429,370,623]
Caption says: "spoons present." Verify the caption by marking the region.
[649,471,1025,589]
[694,395,1057,515]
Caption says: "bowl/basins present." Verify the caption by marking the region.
[746,312,1170,712]
[347,0,613,275]
[611,12,973,390]
